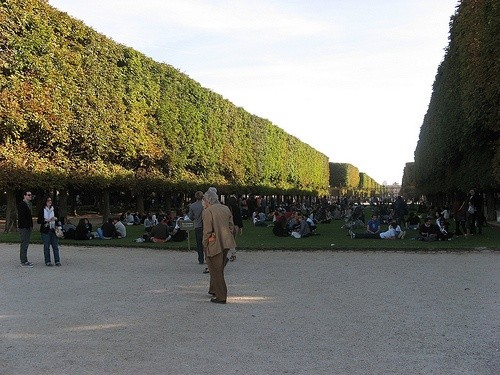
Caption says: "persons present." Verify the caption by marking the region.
[313,194,335,224]
[368,194,407,228]
[456,188,484,237]
[347,197,365,224]
[339,195,347,215]
[120,211,143,225]
[140,210,190,243]
[36,197,63,267]
[60,218,95,241]
[405,203,452,241]
[238,193,269,227]
[17,191,34,267]
[189,192,206,264]
[96,218,126,240]
[200,187,237,303]
[348,214,406,240]
[270,204,317,237]
[227,194,244,236]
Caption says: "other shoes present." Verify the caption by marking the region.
[21,261,34,268]
[208,291,216,297]
[46,262,53,266]
[211,298,226,304]
[349,229,355,240]
[56,262,61,266]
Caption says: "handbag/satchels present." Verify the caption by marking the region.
[55,228,64,240]
